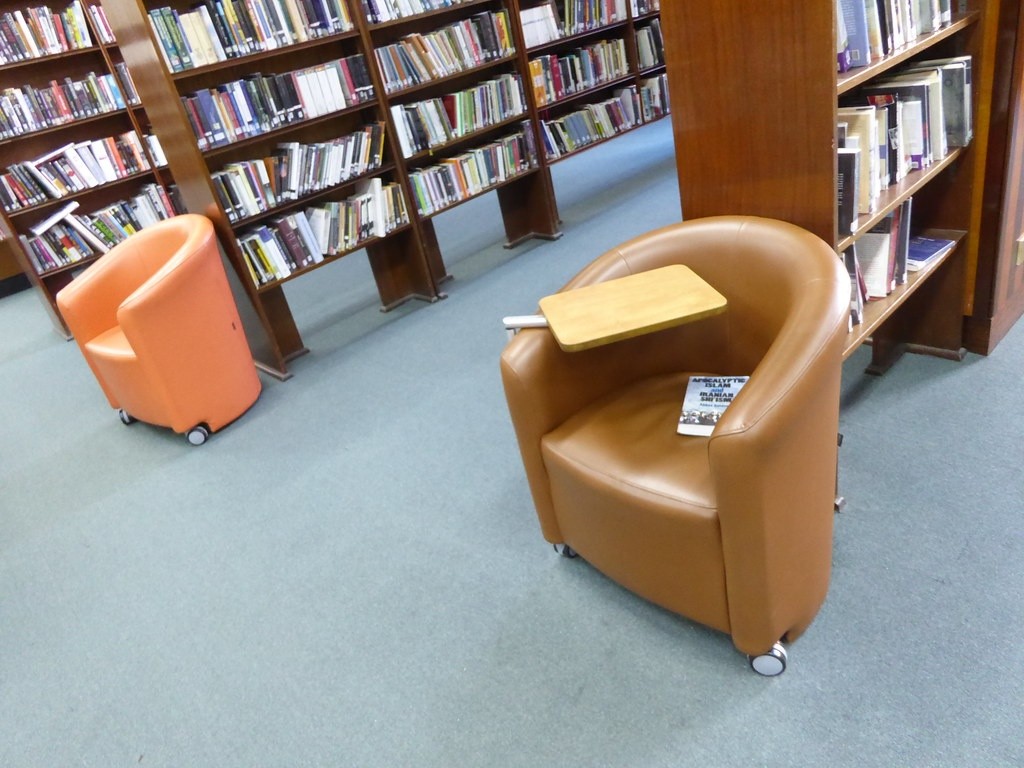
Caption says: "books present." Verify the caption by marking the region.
[1,1,973,437]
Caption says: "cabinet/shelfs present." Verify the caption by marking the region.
[0,0,186,342]
[98,0,671,383]
[658,0,1001,510]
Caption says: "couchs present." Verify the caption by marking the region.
[497,213,852,678]
[56,214,263,448]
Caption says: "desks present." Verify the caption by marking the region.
[504,263,728,353]
